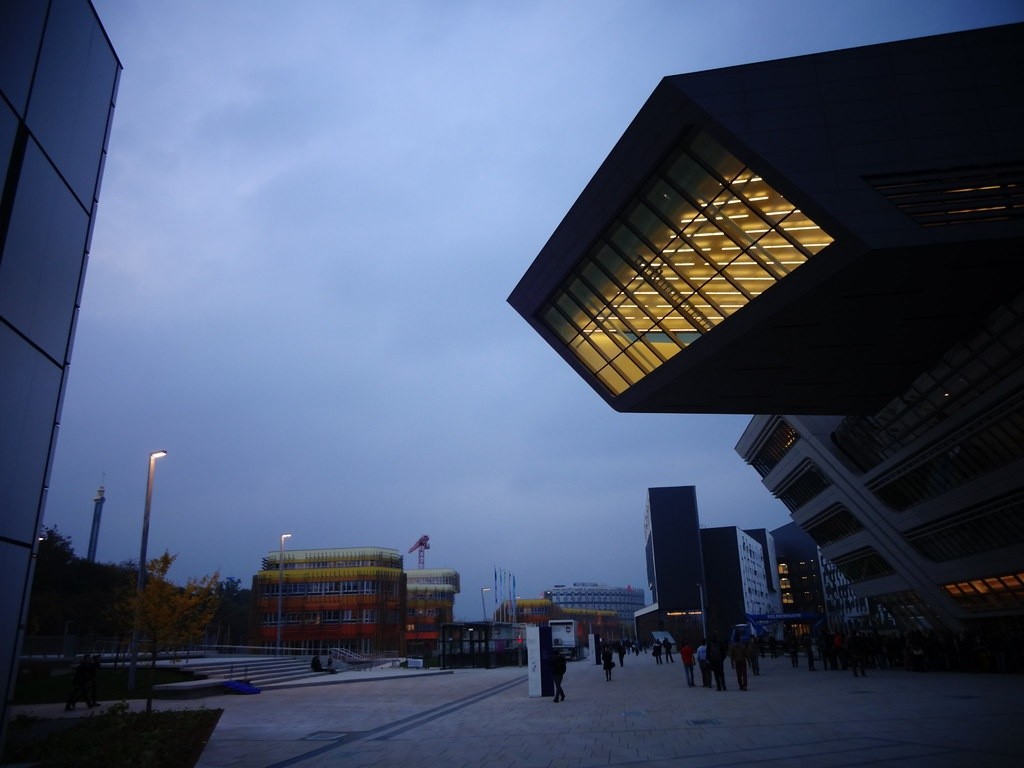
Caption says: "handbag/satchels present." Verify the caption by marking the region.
[611,661,616,668]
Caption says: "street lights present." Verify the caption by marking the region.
[274,532,292,657]
[481,586,492,622]
[124,449,169,698]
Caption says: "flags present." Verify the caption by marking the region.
[634,587,638,595]
[626,585,633,593]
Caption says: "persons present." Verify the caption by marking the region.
[696,636,709,688]
[601,646,612,683]
[729,635,750,691]
[757,610,1024,680]
[705,630,728,691]
[63,653,102,711]
[680,640,697,688]
[746,633,765,677]
[598,636,675,668]
[311,652,337,674]
[547,649,567,702]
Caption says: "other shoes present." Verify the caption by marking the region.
[554,697,559,702]
[561,695,565,701]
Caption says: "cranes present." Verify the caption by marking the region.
[407,534,432,569]
